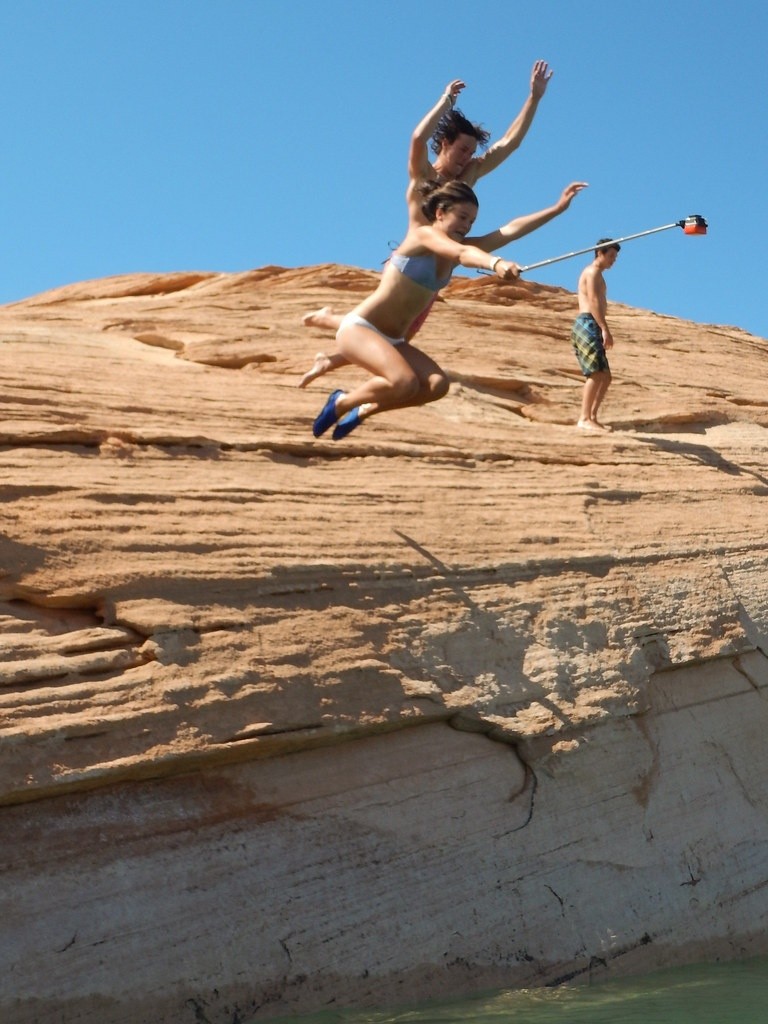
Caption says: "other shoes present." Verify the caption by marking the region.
[313,389,344,438]
[332,407,362,441]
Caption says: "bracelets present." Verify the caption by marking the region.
[441,94,453,107]
[490,256,502,271]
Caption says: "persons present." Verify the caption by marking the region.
[295,59,554,388]
[311,179,590,441]
[570,238,621,434]
